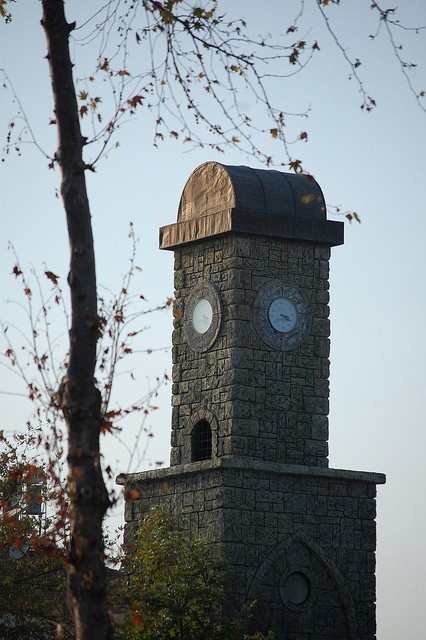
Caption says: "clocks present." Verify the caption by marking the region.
[192,299,213,335]
[268,297,300,334]
